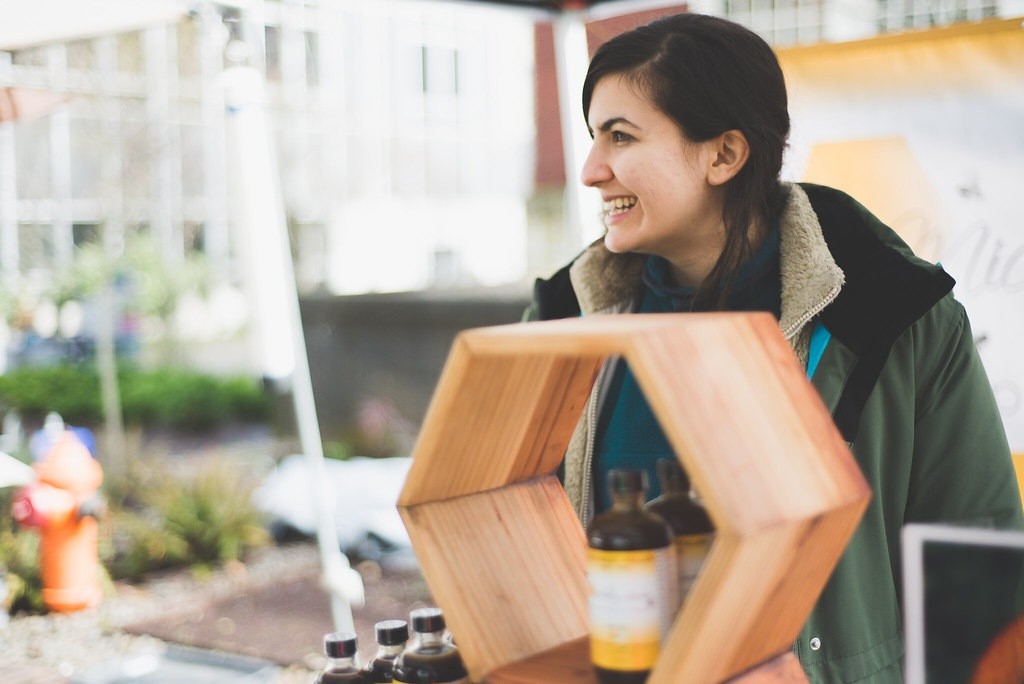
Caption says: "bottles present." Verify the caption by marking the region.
[585,458,716,684]
[314,608,470,684]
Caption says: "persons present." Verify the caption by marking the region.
[519,14,1024,683]
